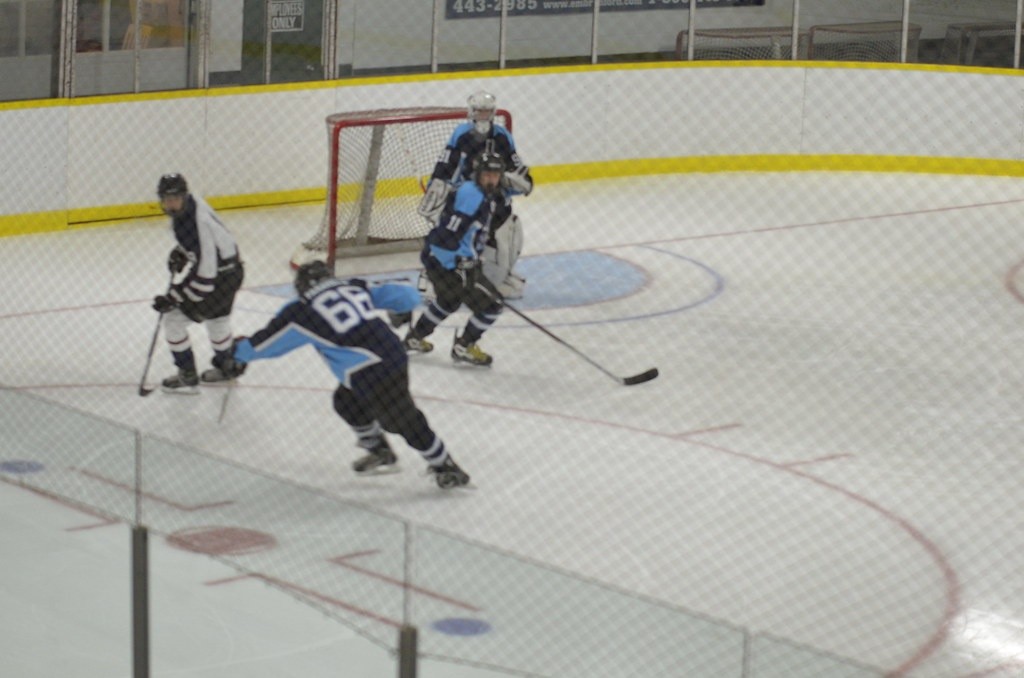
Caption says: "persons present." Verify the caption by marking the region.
[220,261,475,488]
[402,153,510,366]
[152,174,244,391]
[421,90,533,299]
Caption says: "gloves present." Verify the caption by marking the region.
[152,289,183,313]
[167,246,189,273]
[447,255,476,294]
[220,347,247,378]
[389,311,412,328]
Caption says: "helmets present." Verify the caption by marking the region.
[466,91,497,109]
[155,173,188,194]
[471,152,507,171]
[295,260,330,292]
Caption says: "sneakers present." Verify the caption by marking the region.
[199,344,238,386]
[426,453,470,489]
[158,346,200,394]
[450,327,493,369]
[352,432,398,471]
[404,319,433,352]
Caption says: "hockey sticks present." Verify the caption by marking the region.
[136,274,174,396]
[503,299,659,386]
